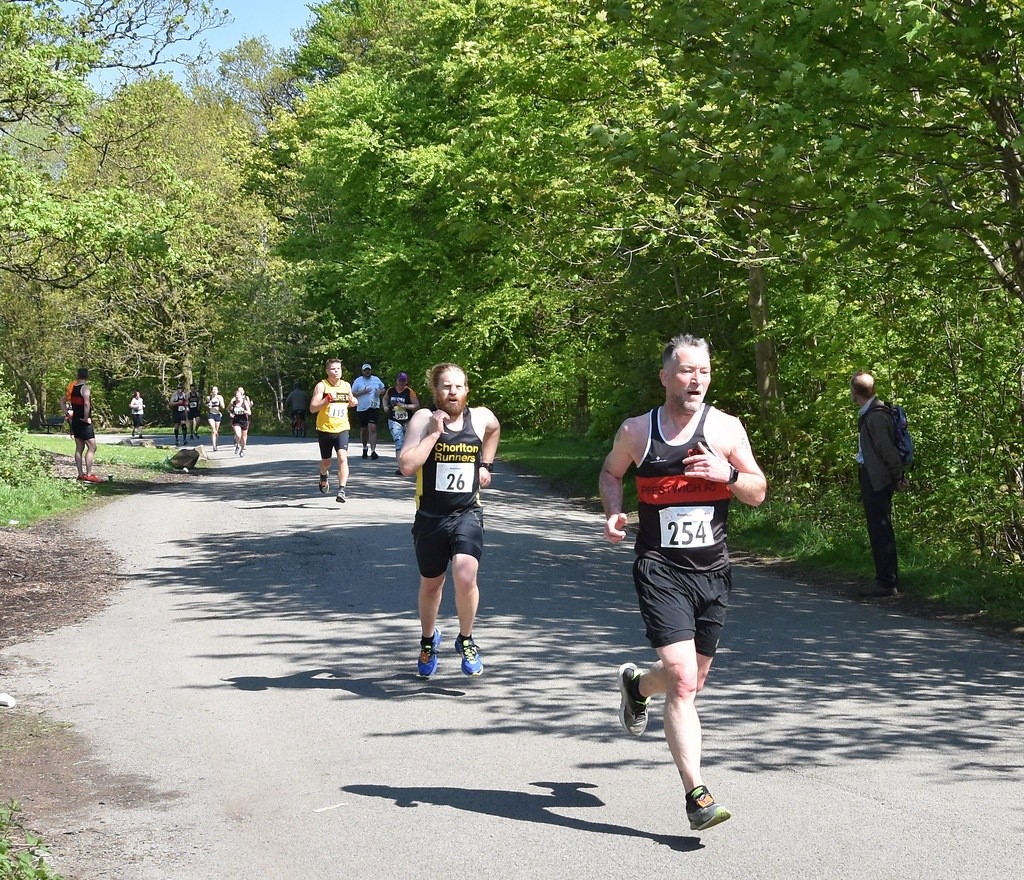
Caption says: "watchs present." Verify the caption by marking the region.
[480,463,493,472]
[724,466,738,485]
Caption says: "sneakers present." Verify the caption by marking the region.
[318,470,329,494]
[685,790,731,831]
[455,635,483,676]
[417,626,441,678]
[84,474,104,484]
[77,474,88,480]
[336,490,347,503]
[618,662,649,738]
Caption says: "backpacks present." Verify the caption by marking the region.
[858,401,914,466]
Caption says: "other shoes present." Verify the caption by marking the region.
[132,431,134,436]
[362,449,368,459]
[240,450,244,457]
[194,433,199,439]
[371,451,378,460]
[139,435,143,439]
[234,446,240,454]
[174,441,180,446]
[182,440,188,446]
[190,435,194,440]
[858,582,897,596]
[395,469,403,475]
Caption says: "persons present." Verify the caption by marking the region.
[286,383,308,428]
[170,385,189,446]
[398,361,501,679]
[61,368,103,484]
[850,373,910,597]
[185,384,203,440]
[65,406,75,438]
[230,387,253,450]
[352,364,386,460]
[599,336,766,830]
[226,391,251,457]
[310,359,358,503]
[205,385,225,451]
[129,392,144,439]
[382,373,420,475]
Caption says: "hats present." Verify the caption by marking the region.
[294,382,301,387]
[397,373,408,381]
[362,364,371,370]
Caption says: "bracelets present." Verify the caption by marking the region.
[66,413,69,416]
[404,404,408,408]
